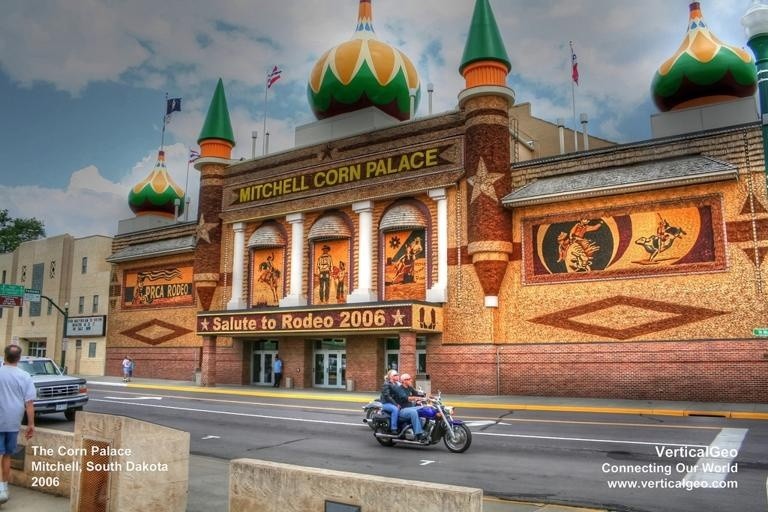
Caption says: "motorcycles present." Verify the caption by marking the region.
[363,385,472,453]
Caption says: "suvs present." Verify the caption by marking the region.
[0,354,87,420]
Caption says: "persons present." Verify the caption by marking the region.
[271,353,283,386]
[386,257,398,285]
[336,260,346,299]
[396,373,429,441]
[556,232,581,263]
[122,355,131,382]
[0,344,36,504]
[569,218,603,257]
[382,368,401,435]
[266,251,275,269]
[317,244,334,303]
[128,356,133,381]
[398,245,416,284]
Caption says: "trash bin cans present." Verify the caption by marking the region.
[195,367,202,387]
[415,374,432,396]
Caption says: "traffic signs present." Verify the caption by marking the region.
[1,283,42,306]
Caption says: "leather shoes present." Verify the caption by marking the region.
[418,432,430,441]
[390,430,399,435]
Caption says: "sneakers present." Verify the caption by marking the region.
[0,481,9,503]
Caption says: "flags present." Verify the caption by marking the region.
[572,47,581,85]
[167,97,184,114]
[267,65,282,88]
